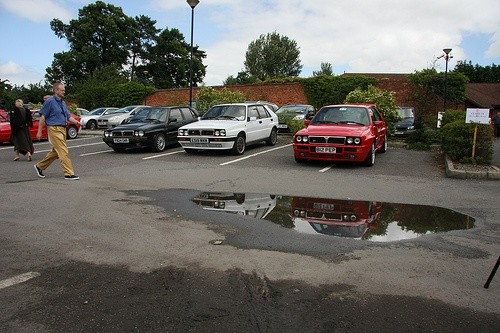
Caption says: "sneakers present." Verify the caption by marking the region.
[65,175,79,180]
[34,164,45,177]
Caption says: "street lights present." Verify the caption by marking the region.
[443,48,453,109]
[186,0,199,107]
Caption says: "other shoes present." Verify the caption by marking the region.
[27,152,32,161]
[13,157,20,161]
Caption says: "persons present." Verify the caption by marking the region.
[10,99,34,161]
[34,83,79,180]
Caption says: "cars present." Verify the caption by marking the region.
[193,192,281,220]
[272,104,317,134]
[176,102,280,156]
[96,105,151,130]
[292,103,387,168]
[291,197,383,241]
[103,105,200,153]
[74,108,89,116]
[390,106,424,136]
[0,109,82,143]
[79,105,120,131]
